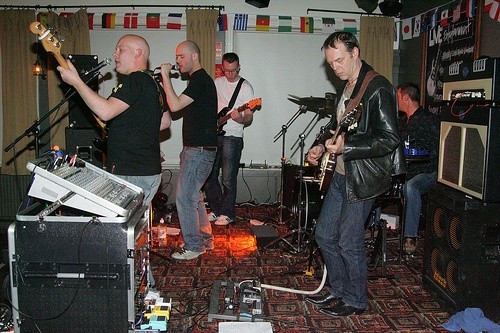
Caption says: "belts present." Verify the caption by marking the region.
[184,146,217,151]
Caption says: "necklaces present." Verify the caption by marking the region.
[345,78,358,87]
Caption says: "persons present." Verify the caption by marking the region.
[204,53,255,225]
[367,82,439,271]
[159,40,217,260]
[57,35,171,286]
[305,30,400,318]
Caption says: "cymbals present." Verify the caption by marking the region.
[287,94,335,115]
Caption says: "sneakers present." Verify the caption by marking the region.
[213,215,236,226]
[170,245,206,262]
[207,212,220,222]
[178,236,215,251]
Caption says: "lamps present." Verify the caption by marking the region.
[33,51,48,80]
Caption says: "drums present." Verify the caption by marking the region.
[290,178,324,242]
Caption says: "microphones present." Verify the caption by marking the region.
[156,65,180,70]
[84,58,113,76]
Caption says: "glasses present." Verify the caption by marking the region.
[222,66,240,73]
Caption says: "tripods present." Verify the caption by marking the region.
[262,105,328,258]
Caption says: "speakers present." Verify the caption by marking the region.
[283,163,326,230]
[437,100,500,204]
[68,54,98,129]
[421,183,500,323]
[65,126,104,170]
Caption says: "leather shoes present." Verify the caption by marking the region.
[318,299,366,318]
[304,292,342,307]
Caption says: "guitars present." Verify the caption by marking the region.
[217,98,262,137]
[319,106,359,193]
[28,16,107,155]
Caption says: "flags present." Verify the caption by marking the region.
[217,14,357,35]
[401,0,500,40]
[38,13,184,31]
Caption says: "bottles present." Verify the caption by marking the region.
[157,217,166,242]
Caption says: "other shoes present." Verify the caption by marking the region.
[402,237,417,255]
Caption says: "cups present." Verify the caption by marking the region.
[158,237,167,247]
